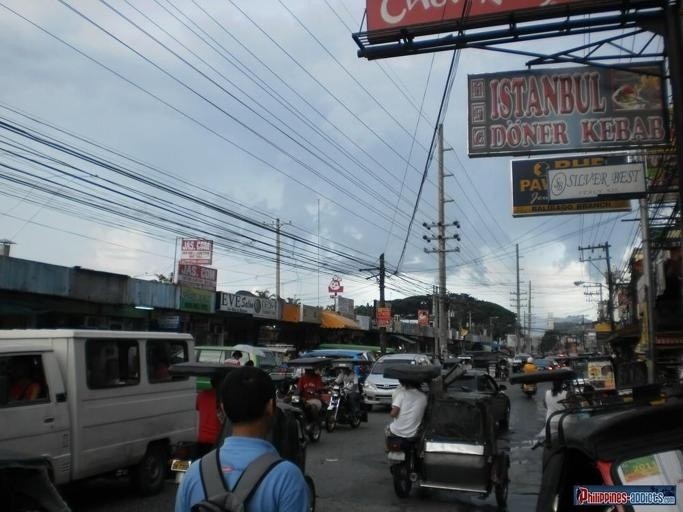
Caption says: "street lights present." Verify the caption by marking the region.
[573,280,610,291]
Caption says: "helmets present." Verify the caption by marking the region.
[527,357,534,364]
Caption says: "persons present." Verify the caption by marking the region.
[579,384,604,407]
[173,364,311,512]
[540,378,569,423]
[327,363,361,414]
[193,366,230,455]
[294,367,326,426]
[222,350,242,366]
[520,356,538,389]
[384,380,429,453]
[559,391,589,424]
[389,378,405,402]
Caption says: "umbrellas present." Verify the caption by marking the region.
[232,343,265,358]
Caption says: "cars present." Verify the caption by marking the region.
[0,328,683,512]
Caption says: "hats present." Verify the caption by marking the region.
[333,363,350,371]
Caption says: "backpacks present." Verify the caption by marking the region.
[190,446,288,512]
